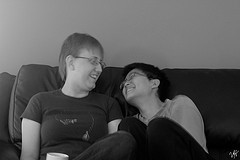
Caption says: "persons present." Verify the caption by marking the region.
[116,63,214,159]
[19,33,135,160]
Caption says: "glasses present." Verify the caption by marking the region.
[120,72,148,89]
[75,57,106,69]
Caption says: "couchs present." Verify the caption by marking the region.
[0,63,240,160]
